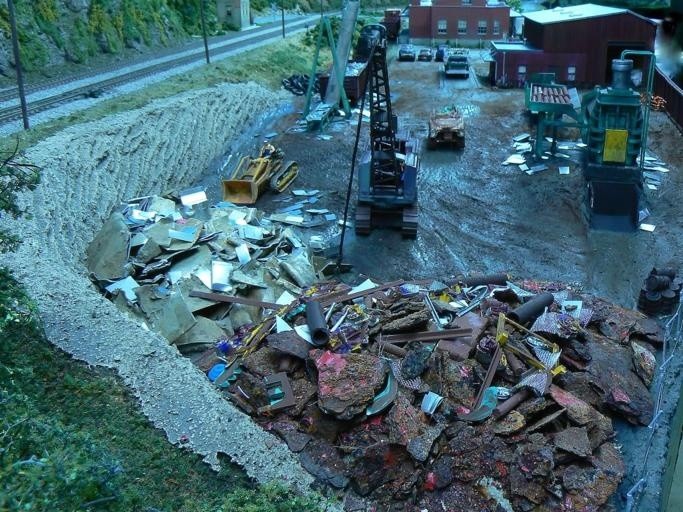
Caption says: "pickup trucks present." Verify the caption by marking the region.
[444,55,470,79]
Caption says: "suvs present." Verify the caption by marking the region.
[429,106,465,151]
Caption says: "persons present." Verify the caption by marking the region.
[260,141,276,159]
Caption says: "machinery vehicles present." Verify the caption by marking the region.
[220,154,299,206]
[354,24,419,239]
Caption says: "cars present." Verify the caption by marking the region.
[398,43,449,62]
